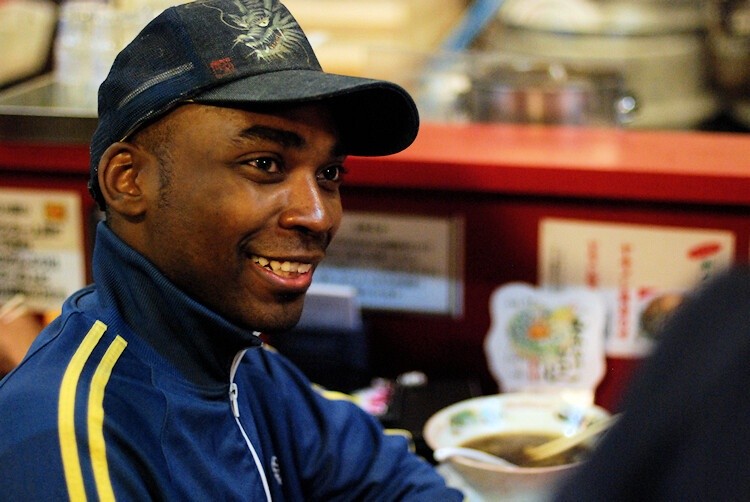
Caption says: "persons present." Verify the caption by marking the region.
[0,1,463,502]
[546,266,750,502]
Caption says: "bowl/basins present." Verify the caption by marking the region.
[424,390,613,502]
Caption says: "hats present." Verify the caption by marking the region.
[89,0,421,200]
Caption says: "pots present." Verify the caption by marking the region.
[459,62,639,129]
